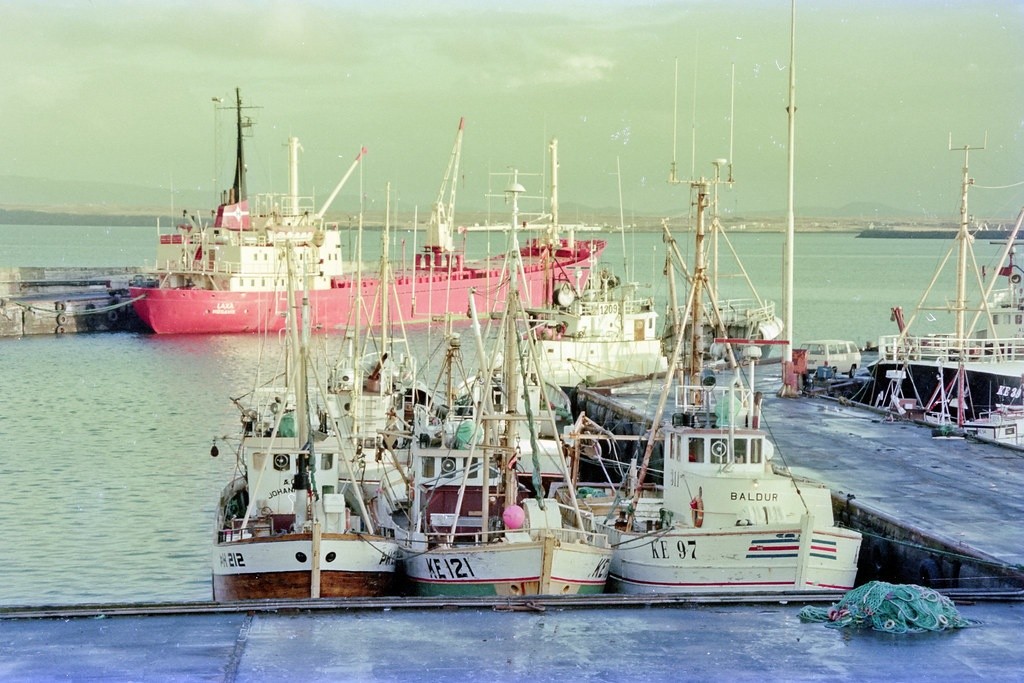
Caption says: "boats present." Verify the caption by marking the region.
[128,85,609,336]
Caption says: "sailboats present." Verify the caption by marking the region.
[212,9,1024,606]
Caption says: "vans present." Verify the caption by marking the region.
[802,339,861,378]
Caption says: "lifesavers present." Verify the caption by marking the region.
[85,304,97,328]
[54,301,68,336]
[691,496,706,529]
[107,296,119,322]
